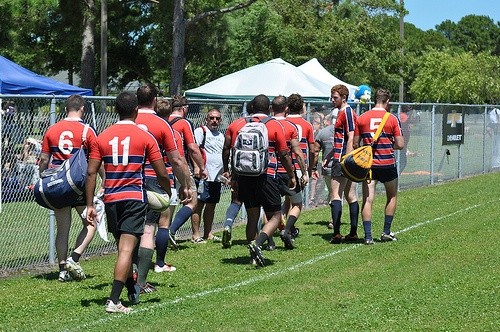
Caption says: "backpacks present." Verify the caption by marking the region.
[231,116,269,176]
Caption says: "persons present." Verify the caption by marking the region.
[39,94,104,282]
[352,88,404,245]
[0,92,232,273]
[321,84,360,244]
[86,91,172,313]
[218,104,419,269]
[411,101,500,169]
[135,83,188,298]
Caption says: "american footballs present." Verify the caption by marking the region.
[145,178,170,212]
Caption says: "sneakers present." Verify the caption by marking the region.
[247,240,266,267]
[135,281,156,293]
[280,230,295,249]
[328,234,342,244]
[106,298,130,313]
[381,233,398,241]
[291,228,299,239]
[127,284,141,303]
[364,238,374,244]
[63,256,87,280]
[58,270,73,281]
[154,264,177,273]
[266,243,275,250]
[222,227,232,248]
[341,233,358,242]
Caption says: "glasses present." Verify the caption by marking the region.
[208,117,221,120]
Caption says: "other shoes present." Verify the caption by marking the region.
[207,236,221,241]
[168,230,178,248]
[191,235,206,244]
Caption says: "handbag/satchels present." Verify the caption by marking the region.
[341,146,373,183]
[34,149,90,211]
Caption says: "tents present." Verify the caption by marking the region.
[0,55,98,130]
[183,57,358,122]
[296,58,371,208]
